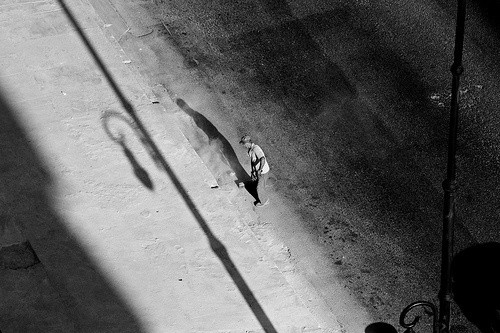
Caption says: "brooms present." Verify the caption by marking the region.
[237,175,265,189]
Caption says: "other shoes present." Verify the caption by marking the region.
[255,198,270,207]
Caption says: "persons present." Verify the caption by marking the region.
[239,135,270,207]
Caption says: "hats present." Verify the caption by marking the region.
[241,135,251,144]
